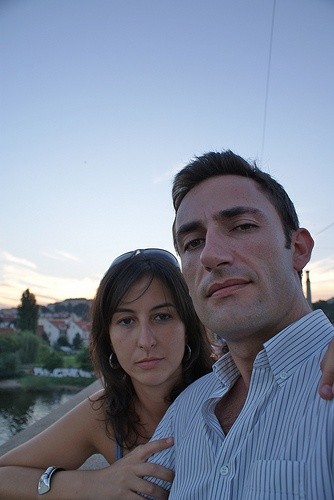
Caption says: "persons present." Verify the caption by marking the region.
[137,151,334,500]
[0,249,334,500]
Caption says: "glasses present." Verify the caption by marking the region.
[111,248,180,268]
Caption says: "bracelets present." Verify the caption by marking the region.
[38,465,68,495]
